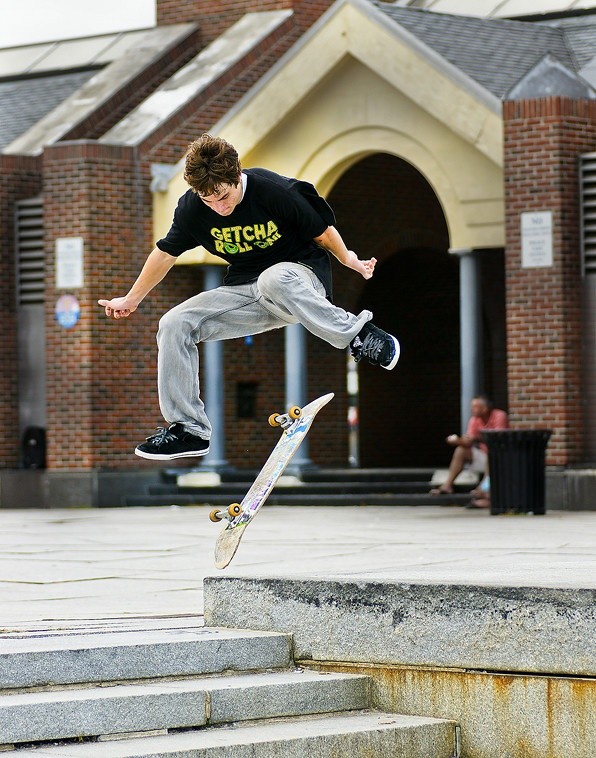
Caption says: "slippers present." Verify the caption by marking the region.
[429,487,452,495]
[469,497,490,510]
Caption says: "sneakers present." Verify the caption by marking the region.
[134,422,210,460]
[350,322,400,371]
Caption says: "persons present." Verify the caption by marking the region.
[98,133,401,462]
[429,396,509,495]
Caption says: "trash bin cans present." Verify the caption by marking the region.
[483,428,556,516]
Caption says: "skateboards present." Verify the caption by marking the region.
[208,392,336,570]
